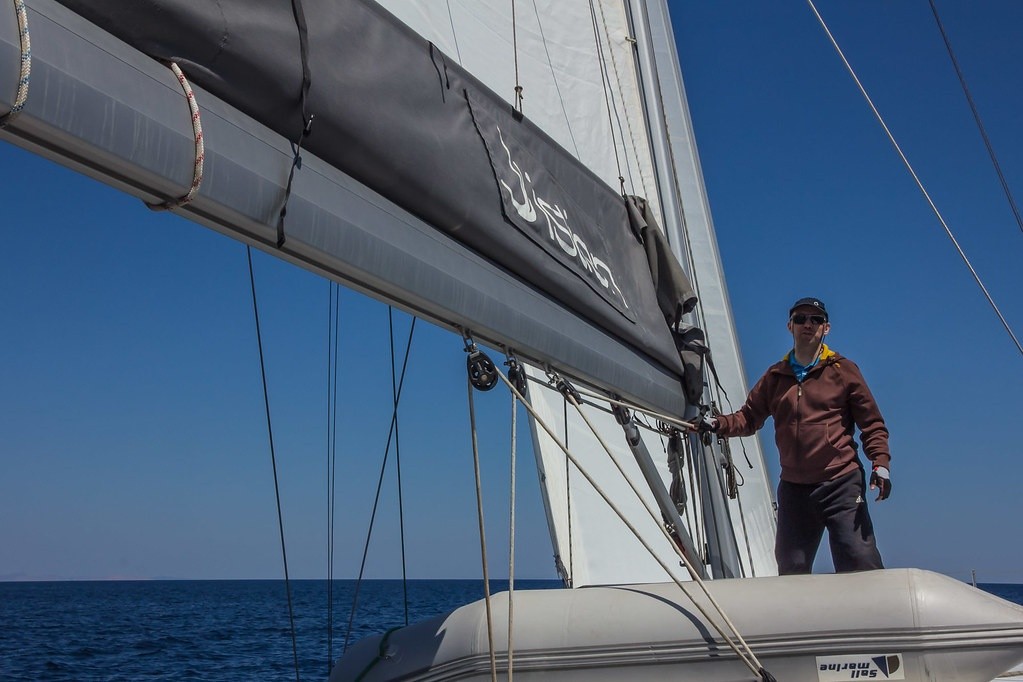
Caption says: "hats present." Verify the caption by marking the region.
[789,297,829,323]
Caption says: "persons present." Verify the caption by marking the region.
[687,298,893,577]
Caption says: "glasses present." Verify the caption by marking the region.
[790,314,825,325]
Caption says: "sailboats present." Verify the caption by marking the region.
[0,0,1023,682]
